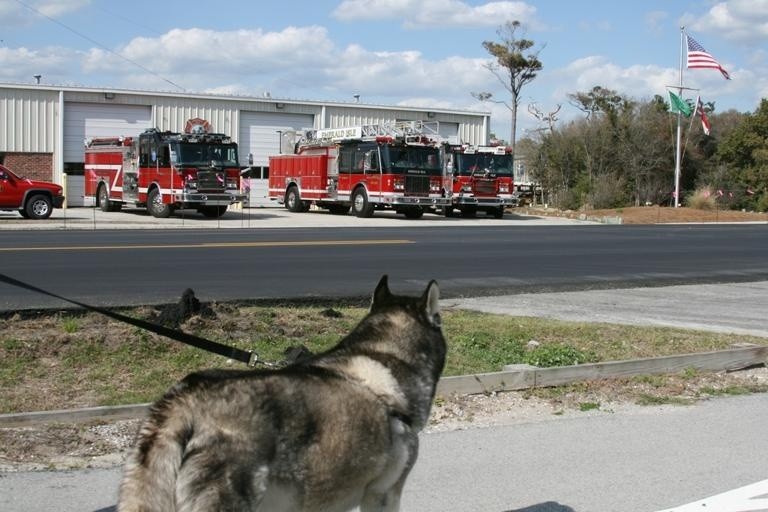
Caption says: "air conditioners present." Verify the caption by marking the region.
[114,275,447,512]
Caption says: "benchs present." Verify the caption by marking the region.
[683,30,733,81]
[667,90,691,118]
[695,96,711,136]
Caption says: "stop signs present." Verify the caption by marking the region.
[429,139,519,220]
[83,129,242,219]
[268,119,455,220]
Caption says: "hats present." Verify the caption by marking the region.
[0,163,65,219]
